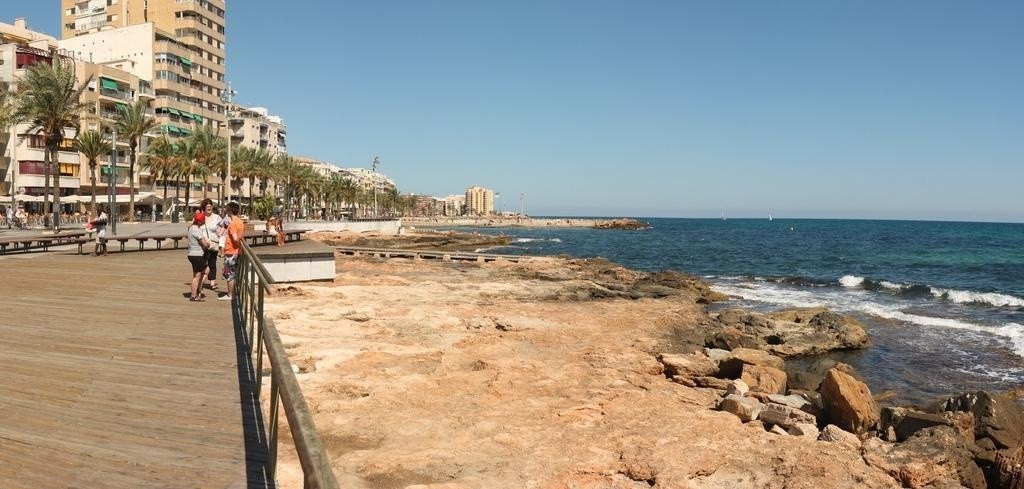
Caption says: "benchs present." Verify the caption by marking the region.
[0,232,188,257]
[243,228,314,247]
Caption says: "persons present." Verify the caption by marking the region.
[5,204,13,229]
[214,202,243,301]
[200,198,224,289]
[266,216,285,246]
[85,202,108,257]
[216,206,230,258]
[14,205,28,230]
[186,214,210,301]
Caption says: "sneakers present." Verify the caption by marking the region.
[218,293,235,301]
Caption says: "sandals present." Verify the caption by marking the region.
[209,284,218,290]
[190,294,205,302]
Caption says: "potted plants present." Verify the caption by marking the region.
[183,211,193,225]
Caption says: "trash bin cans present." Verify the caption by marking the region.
[172,211,179,223]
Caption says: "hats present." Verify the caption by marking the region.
[194,212,204,222]
